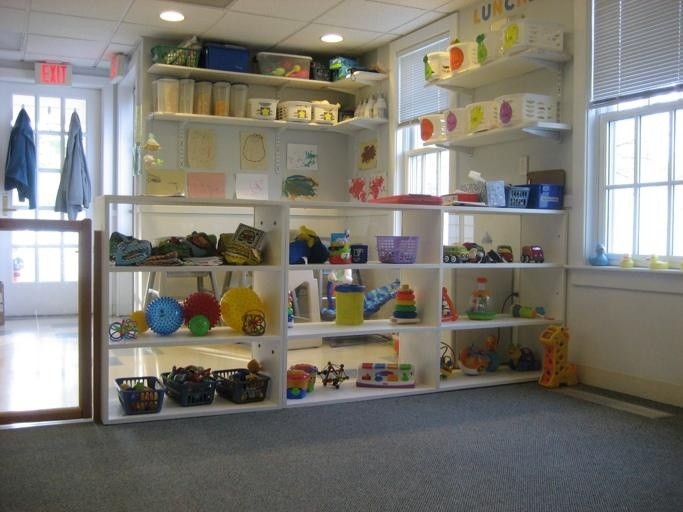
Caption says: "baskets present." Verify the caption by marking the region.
[247,97,338,123]
[504,185,531,208]
[376,235,419,264]
[114,376,165,415]
[150,45,202,67]
[417,92,558,142]
[160,372,216,407]
[212,368,271,404]
[421,18,565,82]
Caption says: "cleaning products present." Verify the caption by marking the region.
[354,95,386,119]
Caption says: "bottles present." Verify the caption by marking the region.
[354,93,387,118]
[150,76,249,117]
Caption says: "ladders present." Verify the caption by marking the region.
[142,272,222,312]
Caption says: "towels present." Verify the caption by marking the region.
[3,109,37,208]
[55,112,92,220]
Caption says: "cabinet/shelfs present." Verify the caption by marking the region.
[424,50,573,155]
[131,36,401,198]
[95,198,567,424]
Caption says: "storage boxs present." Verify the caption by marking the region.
[256,50,313,80]
[514,170,567,209]
[201,44,249,72]
